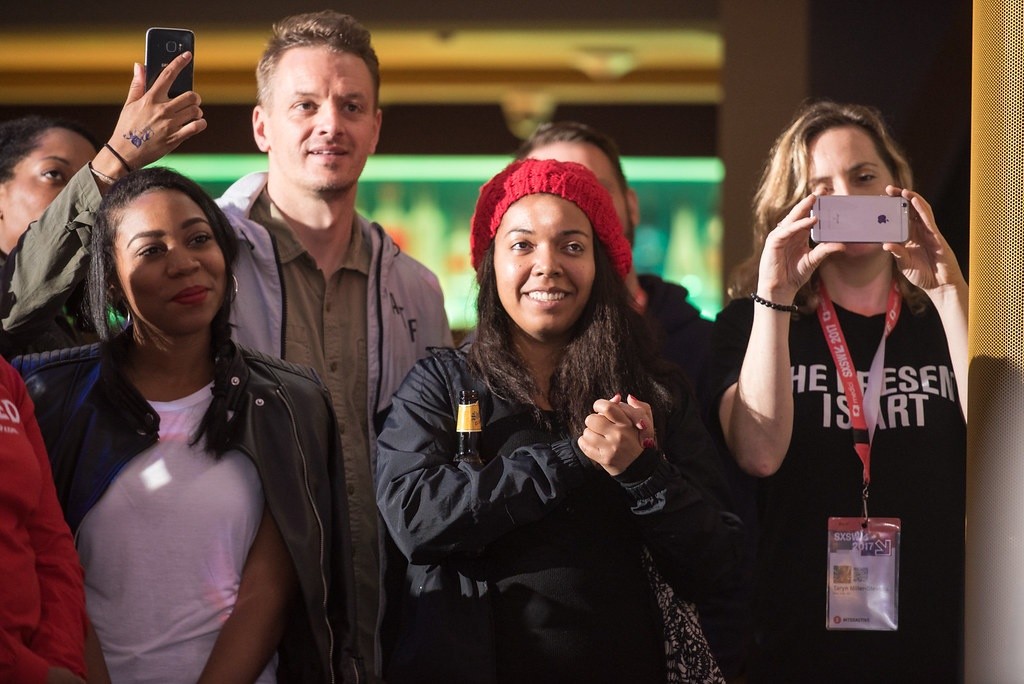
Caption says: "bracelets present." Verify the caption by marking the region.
[104,143,134,173]
[751,293,799,312]
[90,168,117,183]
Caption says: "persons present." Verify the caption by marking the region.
[0,51,207,364]
[0,357,88,684]
[212,10,457,684]
[378,126,714,683]
[715,102,969,683]
[13,168,356,683]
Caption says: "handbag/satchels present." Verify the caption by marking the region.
[652,582,723,684]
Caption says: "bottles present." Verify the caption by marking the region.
[448,391,487,469]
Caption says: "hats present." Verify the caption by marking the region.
[468,157,633,283]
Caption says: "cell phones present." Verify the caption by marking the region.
[143,27,195,126]
[810,194,910,243]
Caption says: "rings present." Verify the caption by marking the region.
[776,222,785,228]
